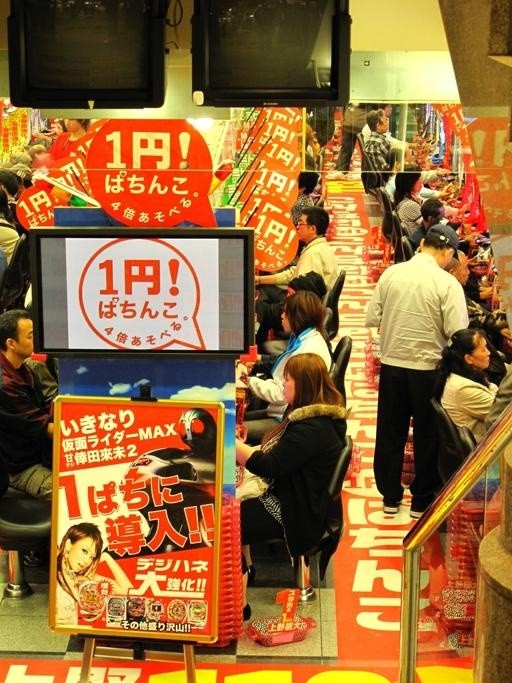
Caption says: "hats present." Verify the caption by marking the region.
[428,224,458,249]
[286,269,328,301]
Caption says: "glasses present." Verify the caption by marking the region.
[296,221,311,227]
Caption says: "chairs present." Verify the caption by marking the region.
[355,109,509,526]
[1,111,350,603]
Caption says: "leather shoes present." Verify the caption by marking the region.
[247,564,256,580]
[242,604,252,620]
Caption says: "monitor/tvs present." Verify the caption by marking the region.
[29,227,257,363]
[192,0,350,108]
[7,0,166,109]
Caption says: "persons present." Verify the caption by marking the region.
[326,104,367,179]
[53,520,135,628]
[365,223,469,516]
[0,117,92,506]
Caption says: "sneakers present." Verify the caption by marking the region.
[409,500,426,518]
[382,496,401,514]
[326,171,349,180]
